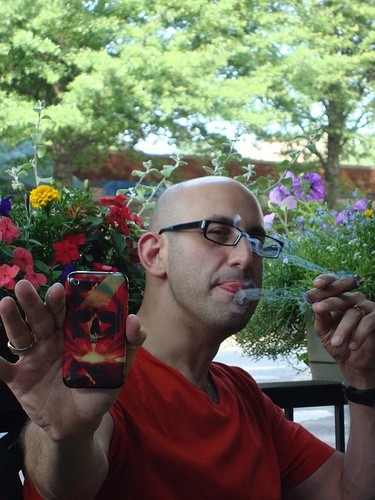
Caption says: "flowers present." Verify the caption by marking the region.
[0,100,190,319]
[201,120,375,360]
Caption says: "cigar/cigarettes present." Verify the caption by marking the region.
[303,272,365,304]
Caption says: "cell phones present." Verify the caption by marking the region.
[62,270,129,389]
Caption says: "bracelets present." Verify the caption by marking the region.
[341,380,375,408]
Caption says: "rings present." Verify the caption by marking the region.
[7,329,38,356]
[354,304,366,317]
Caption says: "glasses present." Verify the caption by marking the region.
[158,219,284,259]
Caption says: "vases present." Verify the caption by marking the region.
[306,308,346,382]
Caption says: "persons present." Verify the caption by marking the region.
[1,176,375,500]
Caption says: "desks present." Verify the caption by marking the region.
[212,347,349,454]
